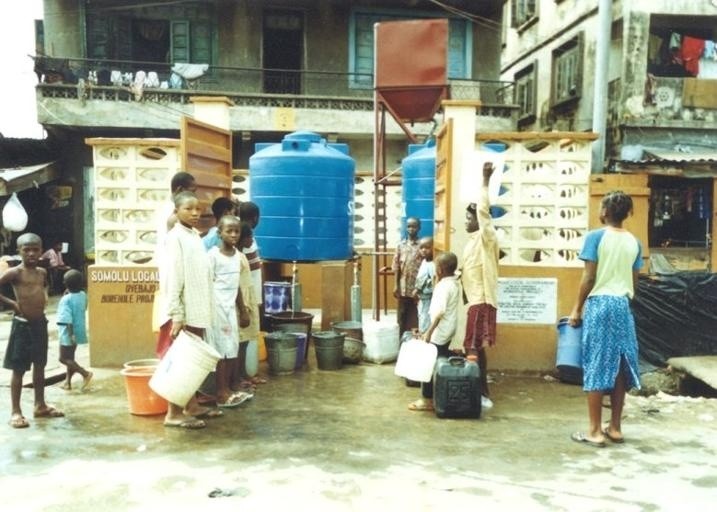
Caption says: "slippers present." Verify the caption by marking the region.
[603,426,624,443]
[163,376,266,428]
[408,400,433,411]
[573,432,604,447]
[9,410,28,428]
[33,409,63,418]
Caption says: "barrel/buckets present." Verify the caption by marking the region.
[120,367,168,415]
[244,337,259,380]
[264,333,298,375]
[270,310,314,358]
[330,321,362,340]
[122,358,159,368]
[288,332,308,370]
[393,337,438,384]
[344,336,366,363]
[556,316,583,374]
[313,331,347,371]
[400,331,421,387]
[147,330,222,407]
[432,355,482,419]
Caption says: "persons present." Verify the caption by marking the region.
[0,233,65,429]
[412,236,437,338]
[392,216,423,359]
[568,190,644,446]
[37,239,72,292]
[459,163,502,407]
[57,267,95,392]
[404,252,463,413]
[152,172,268,430]
